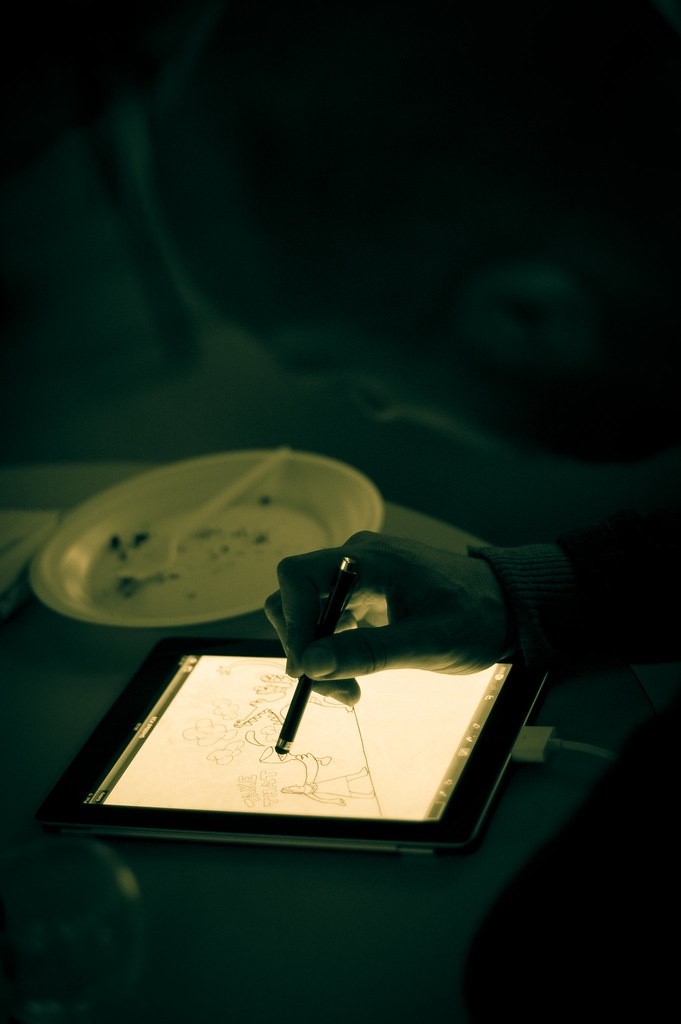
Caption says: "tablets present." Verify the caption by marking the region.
[36,636,551,858]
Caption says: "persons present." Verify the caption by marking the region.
[263,500,681,709]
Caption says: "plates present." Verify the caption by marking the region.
[27,449,384,628]
